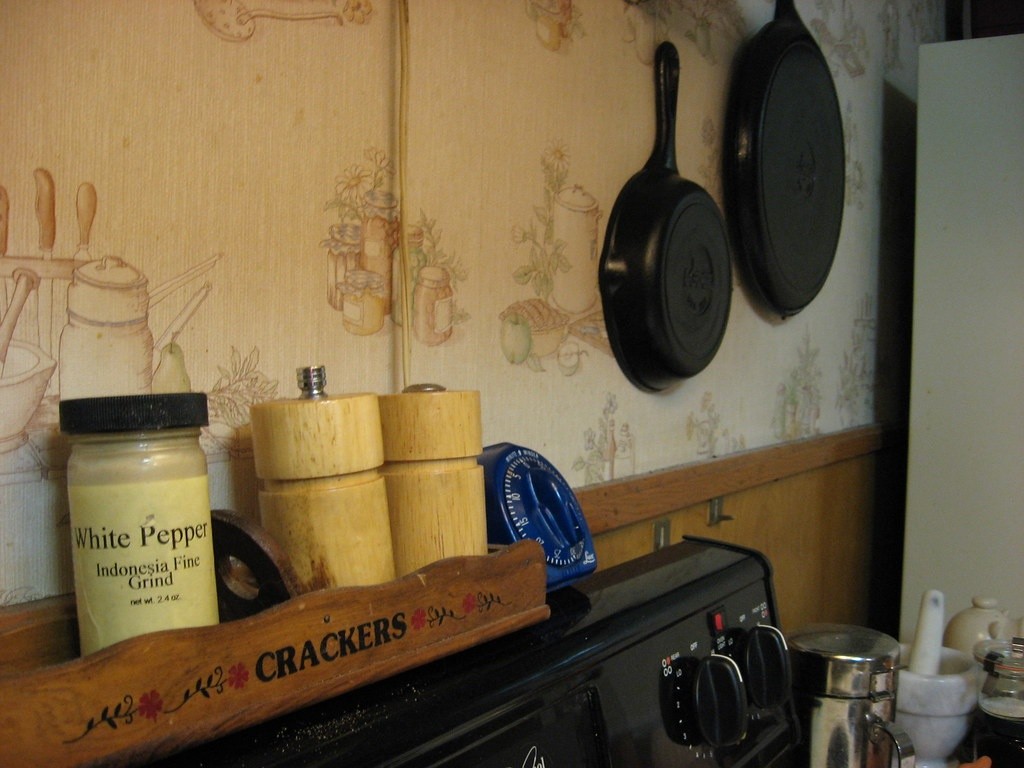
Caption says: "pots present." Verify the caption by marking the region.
[598,40,733,395]
[720,1,846,321]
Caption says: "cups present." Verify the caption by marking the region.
[972,637,1024,768]
[788,620,916,768]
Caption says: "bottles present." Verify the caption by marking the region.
[59,386,220,656]
